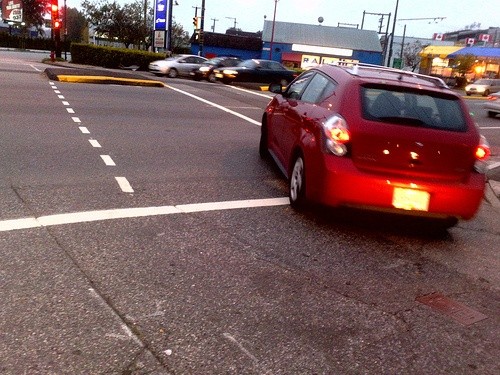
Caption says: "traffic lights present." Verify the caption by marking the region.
[193,17,198,26]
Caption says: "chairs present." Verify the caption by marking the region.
[373,94,402,120]
[404,106,433,124]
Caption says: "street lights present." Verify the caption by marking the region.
[226,16,236,28]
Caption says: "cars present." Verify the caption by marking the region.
[485,91,500,118]
[259,61,492,231]
[464,77,500,97]
[188,56,246,83]
[212,58,303,87]
[148,54,209,79]
[441,71,500,89]
